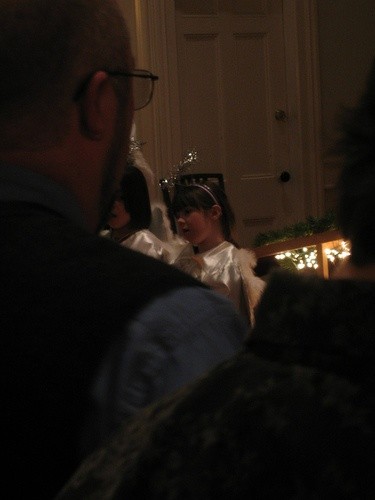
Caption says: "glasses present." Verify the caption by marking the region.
[110,69,159,112]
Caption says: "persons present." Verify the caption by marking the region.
[58,60,375,500]
[0,0,252,499]
